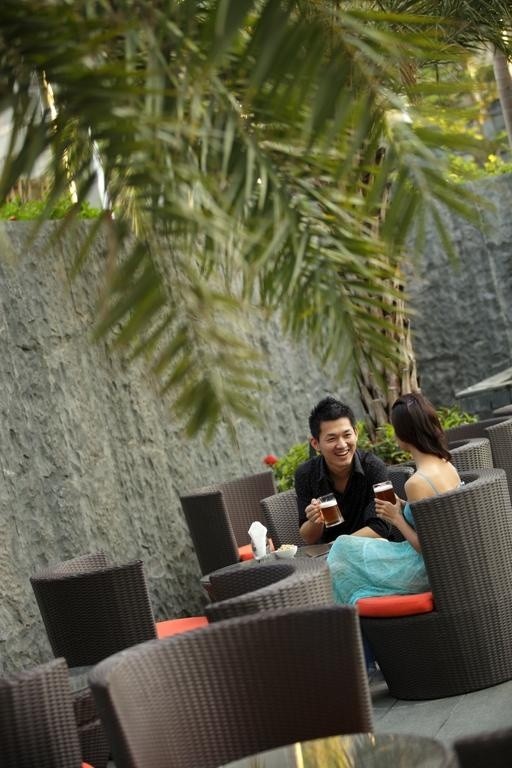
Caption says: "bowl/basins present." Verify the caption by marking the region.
[275,544,298,560]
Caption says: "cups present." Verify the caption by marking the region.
[250,536,272,561]
[316,493,345,528]
[373,480,397,506]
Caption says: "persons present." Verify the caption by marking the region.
[293,395,393,545]
[325,392,464,607]
